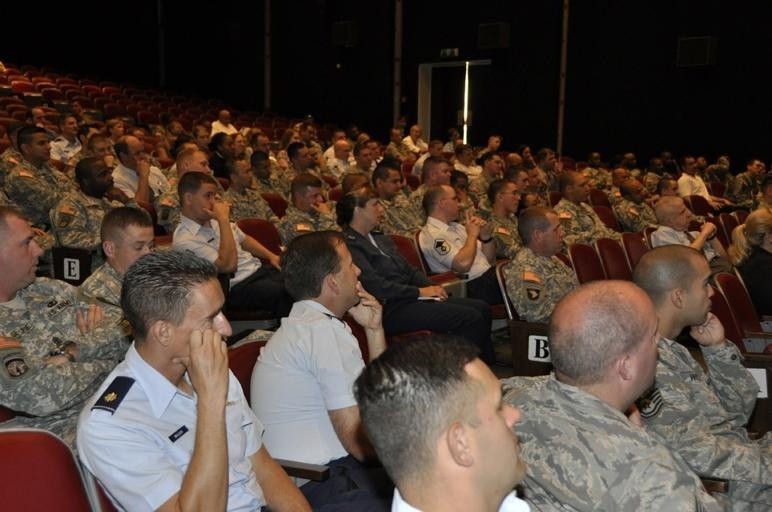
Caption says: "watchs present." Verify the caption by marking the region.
[706,233,717,241]
[50,348,76,363]
[480,236,494,244]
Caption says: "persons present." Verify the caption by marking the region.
[22,106,57,145]
[326,140,355,178]
[731,158,763,207]
[648,195,732,279]
[343,142,374,176]
[250,151,285,196]
[645,156,676,190]
[759,173,772,207]
[241,126,262,140]
[320,130,348,161]
[0,122,29,174]
[418,182,501,299]
[608,168,631,200]
[698,154,711,170]
[126,126,160,166]
[246,133,271,159]
[706,157,729,184]
[371,161,427,236]
[211,109,238,135]
[477,135,502,158]
[656,176,681,196]
[408,156,448,214]
[632,243,772,512]
[350,327,531,512]
[64,124,101,173]
[382,127,416,168]
[50,157,149,273]
[470,152,502,198]
[403,122,426,155]
[505,165,538,208]
[617,151,639,171]
[585,148,613,189]
[153,147,221,234]
[1,127,68,223]
[552,171,621,244]
[453,172,483,226]
[485,179,524,259]
[166,119,184,141]
[0,208,128,444]
[293,114,318,138]
[661,151,674,167]
[677,155,732,211]
[442,127,460,153]
[75,250,311,512]
[278,174,337,241]
[68,101,87,122]
[207,132,234,179]
[336,186,494,355]
[173,170,287,317]
[505,206,582,323]
[222,159,277,232]
[410,139,441,178]
[521,144,537,167]
[279,141,335,184]
[534,148,561,181]
[48,115,82,162]
[106,117,127,145]
[231,132,246,158]
[614,178,655,232]
[495,279,718,512]
[297,123,323,161]
[451,137,464,148]
[453,144,483,175]
[525,162,552,205]
[81,206,157,303]
[364,139,383,166]
[354,133,370,143]
[249,232,385,512]
[110,135,170,209]
[349,121,360,138]
[165,138,208,178]
[342,171,410,236]
[505,153,526,171]
[86,134,119,167]
[190,123,209,149]
[276,127,301,174]
[732,206,772,333]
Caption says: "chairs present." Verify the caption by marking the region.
[735,210,751,231]
[400,161,429,191]
[0,427,96,512]
[225,342,335,482]
[689,220,703,246]
[1,96,23,114]
[11,111,27,124]
[264,112,273,118]
[593,206,622,234]
[36,82,58,98]
[179,102,192,109]
[715,273,772,354]
[169,107,183,119]
[22,64,39,74]
[124,88,139,98]
[103,87,120,93]
[245,111,256,120]
[621,230,649,280]
[27,71,46,80]
[576,162,592,172]
[187,108,203,117]
[111,92,128,103]
[191,98,202,105]
[41,88,62,105]
[116,99,132,107]
[12,80,33,96]
[127,104,139,118]
[734,263,772,332]
[327,185,346,210]
[136,111,156,126]
[261,127,273,137]
[238,116,249,124]
[703,217,731,256]
[199,103,209,110]
[8,74,31,87]
[173,96,185,103]
[344,315,435,376]
[207,108,219,117]
[718,212,740,245]
[236,121,250,130]
[495,260,568,376]
[88,91,104,105]
[72,96,94,113]
[0,75,12,97]
[1,74,7,86]
[689,193,717,222]
[94,96,113,111]
[59,84,79,99]
[0,111,10,121]
[209,99,216,106]
[412,227,510,331]
[0,118,21,138]
[82,85,100,94]
[255,116,268,126]
[80,80,97,90]
[558,156,575,174]
[138,101,154,112]
[546,190,565,212]
[0,137,11,154]
[132,94,149,106]
[104,103,127,119]
[710,181,729,201]
[144,89,160,100]
[159,112,176,126]
[234,217,284,268]
[80,444,121,511]
[66,88,84,107]
[703,286,772,432]
[201,113,215,124]
[644,225,660,249]
[32,106,57,122]
[152,95,168,104]
[262,189,290,222]
[4,68,19,82]
[159,102,175,111]
[383,233,429,281]
[589,188,611,210]
[100,80,113,90]
[55,78,75,89]
[31,75,52,88]
[272,117,285,127]
[567,242,605,294]
[0,125,9,139]
[148,106,163,116]
[595,237,634,284]
[6,104,29,121]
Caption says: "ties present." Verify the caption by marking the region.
[136,172,155,202]
[685,231,704,255]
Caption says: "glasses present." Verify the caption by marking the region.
[499,192,521,196]
[426,196,458,205]
